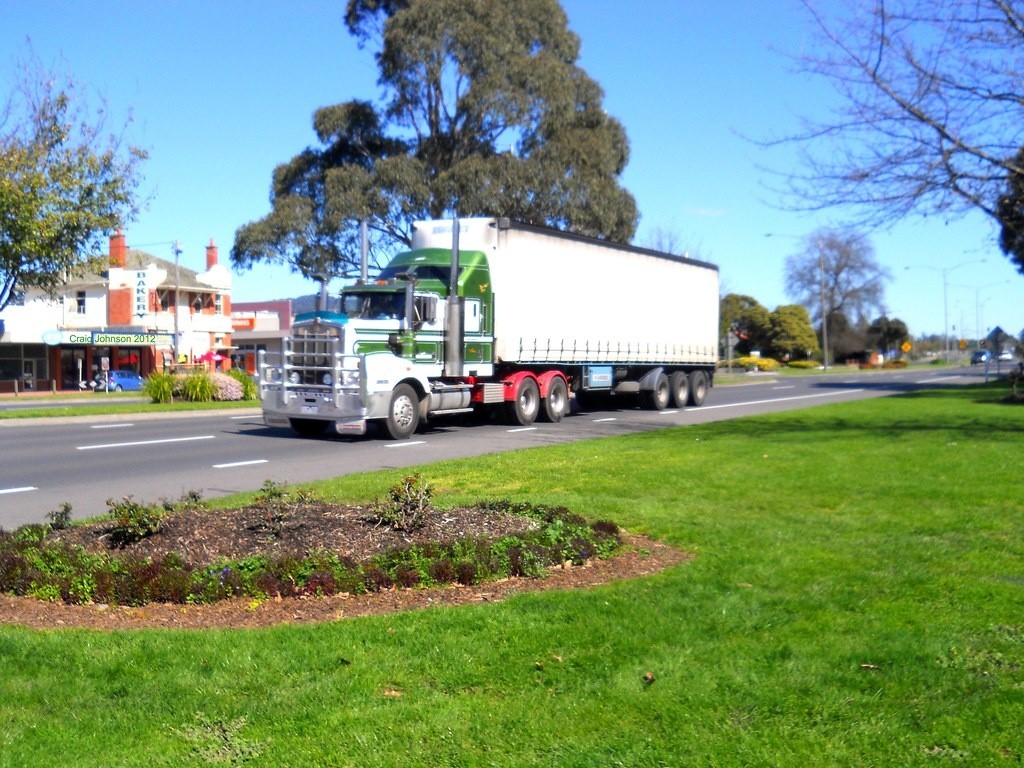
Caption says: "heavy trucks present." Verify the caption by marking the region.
[256,213,721,441]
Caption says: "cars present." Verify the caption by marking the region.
[1000,350,1012,362]
[94,369,145,392]
[972,349,991,365]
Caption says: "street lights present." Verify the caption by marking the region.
[905,263,950,367]
[763,231,829,372]
[174,245,218,365]
